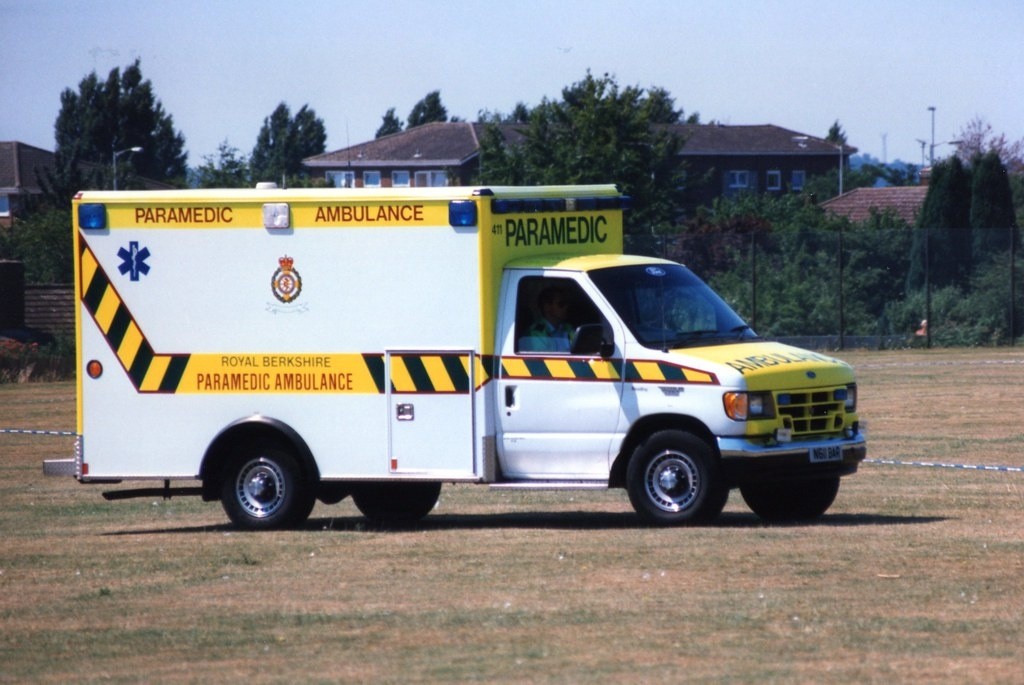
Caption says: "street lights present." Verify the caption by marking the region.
[791,136,844,199]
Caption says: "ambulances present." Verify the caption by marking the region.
[40,181,865,532]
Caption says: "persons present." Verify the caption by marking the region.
[517,278,580,352]
